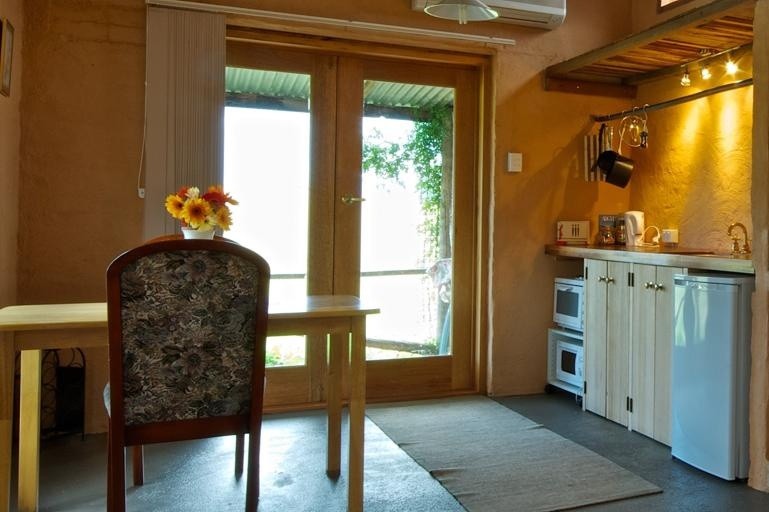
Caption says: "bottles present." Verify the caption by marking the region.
[599,225,624,245]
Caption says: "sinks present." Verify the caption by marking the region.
[694,254,754,260]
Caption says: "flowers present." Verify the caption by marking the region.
[164,184,238,232]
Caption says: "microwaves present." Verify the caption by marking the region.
[552,277,586,333]
[555,337,583,387]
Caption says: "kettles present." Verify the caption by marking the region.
[624,210,645,248]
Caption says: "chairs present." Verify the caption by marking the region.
[102,239,271,512]
[131,233,236,487]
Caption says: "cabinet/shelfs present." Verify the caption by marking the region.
[630,262,688,448]
[581,257,631,430]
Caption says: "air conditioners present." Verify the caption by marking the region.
[411,0,567,30]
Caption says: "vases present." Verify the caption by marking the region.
[180,226,216,240]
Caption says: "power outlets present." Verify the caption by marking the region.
[508,152,523,173]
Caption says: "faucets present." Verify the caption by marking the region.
[727,222,751,255]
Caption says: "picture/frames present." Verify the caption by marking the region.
[0,17,15,98]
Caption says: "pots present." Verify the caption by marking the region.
[595,120,635,187]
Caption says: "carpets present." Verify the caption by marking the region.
[364,395,664,512]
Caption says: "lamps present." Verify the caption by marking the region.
[423,0,499,24]
[680,45,741,86]
